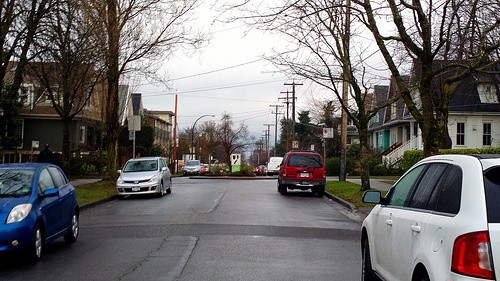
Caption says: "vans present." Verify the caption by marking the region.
[266,156,284,177]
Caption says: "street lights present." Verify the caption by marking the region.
[192,115,215,160]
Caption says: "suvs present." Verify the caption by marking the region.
[277,150,326,198]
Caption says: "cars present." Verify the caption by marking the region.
[116,157,172,201]
[181,159,230,177]
[0,162,81,267]
[255,165,267,176]
[360,154,500,281]
